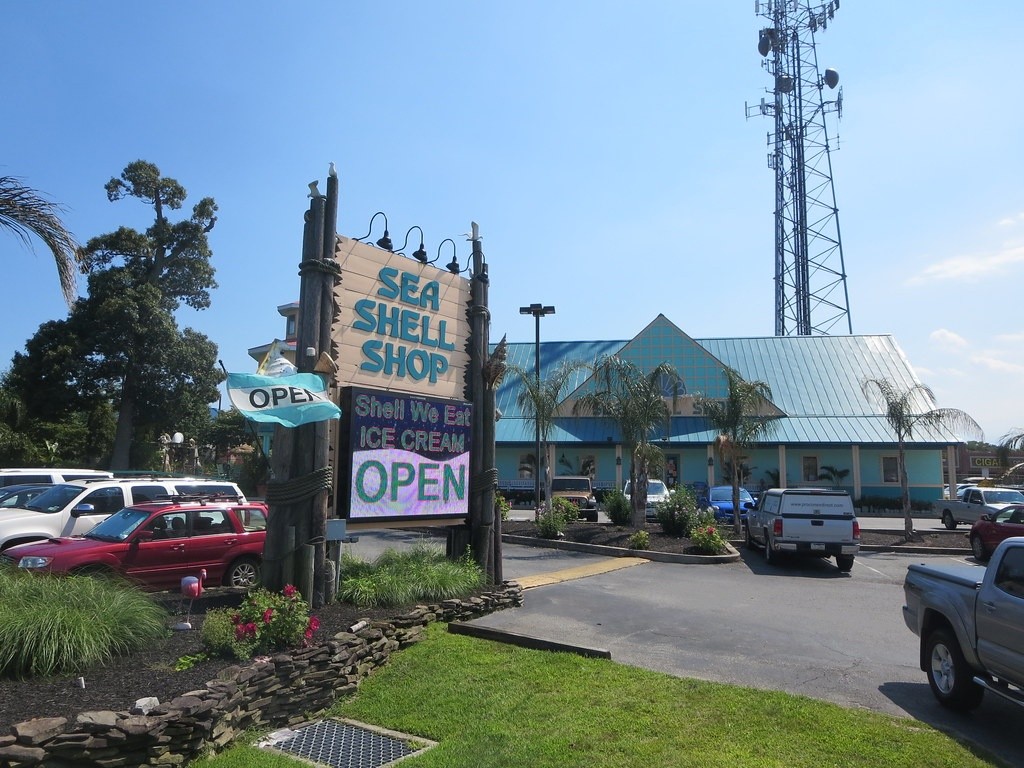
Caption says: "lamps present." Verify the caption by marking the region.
[616,457,622,465]
[708,457,713,465]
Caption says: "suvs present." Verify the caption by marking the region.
[0,494,269,597]
[743,488,860,572]
[552,476,598,522]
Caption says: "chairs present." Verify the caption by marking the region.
[168,517,186,538]
[153,517,167,539]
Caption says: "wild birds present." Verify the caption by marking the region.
[307,162,337,199]
[458,221,483,279]
[180,569,206,623]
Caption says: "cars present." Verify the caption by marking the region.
[623,479,676,523]
[0,468,114,487]
[0,483,119,514]
[700,485,756,524]
[944,484,978,499]
[970,504,1024,560]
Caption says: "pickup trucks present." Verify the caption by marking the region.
[902,537,1024,706]
[936,487,1024,529]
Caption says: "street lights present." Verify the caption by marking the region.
[520,303,555,525]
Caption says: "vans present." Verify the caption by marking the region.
[0,477,248,556]
